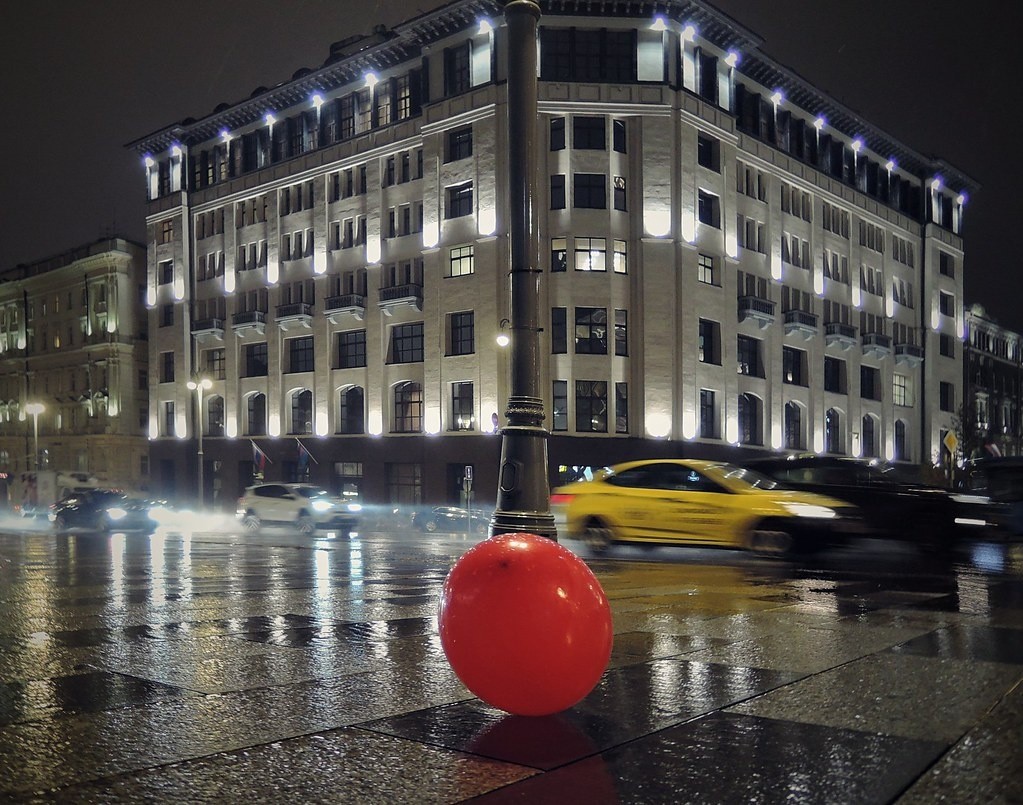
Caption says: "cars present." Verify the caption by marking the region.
[742,451,1022,555]
[410,506,490,534]
[236,481,359,535]
[550,457,857,563]
[49,487,162,532]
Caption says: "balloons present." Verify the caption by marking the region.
[438,532,614,718]
